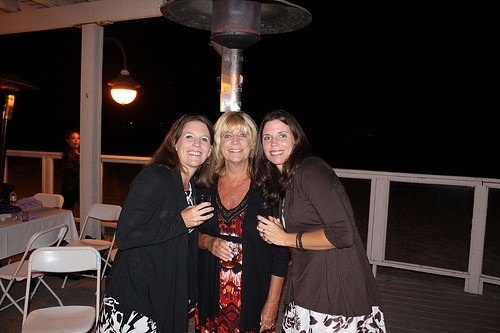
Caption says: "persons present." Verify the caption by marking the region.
[194,111,289,333]
[256,110,387,333]
[59,131,81,217]
[94,113,214,333]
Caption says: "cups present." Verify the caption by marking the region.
[22,210,29,222]
[257,202,273,223]
[193,195,211,209]
[221,247,237,268]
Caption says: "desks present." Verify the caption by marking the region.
[0,207,80,261]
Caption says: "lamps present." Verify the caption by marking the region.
[103,36,142,105]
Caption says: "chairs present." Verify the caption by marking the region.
[0,192,122,333]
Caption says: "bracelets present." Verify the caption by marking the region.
[295,231,306,253]
